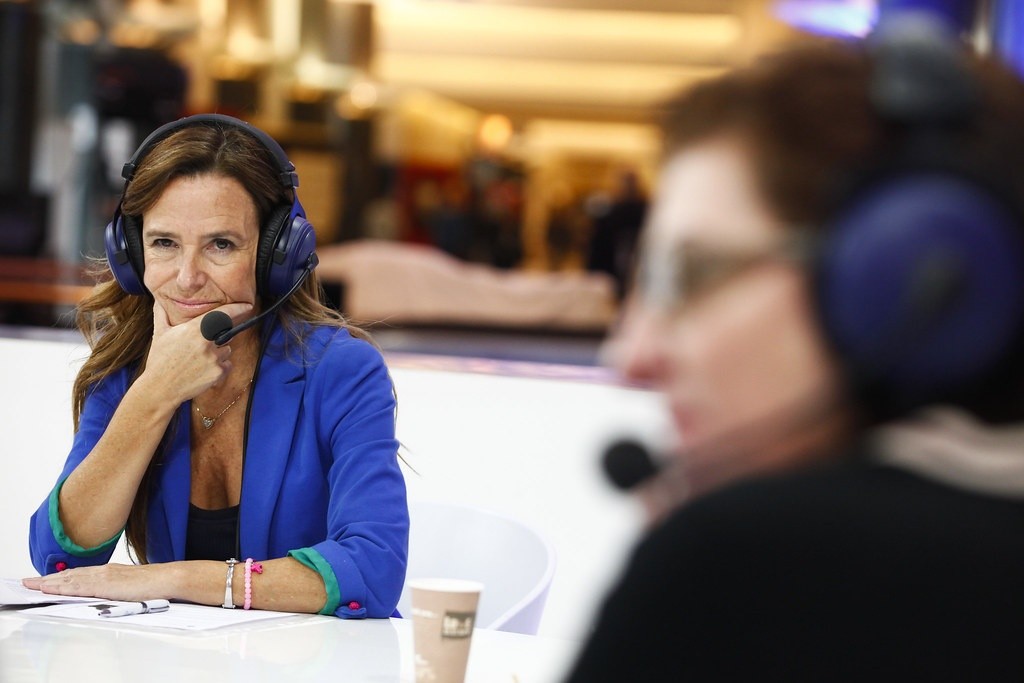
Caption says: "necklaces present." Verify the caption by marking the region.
[193,380,250,431]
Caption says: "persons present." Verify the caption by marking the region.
[22,114,410,619]
[563,34,1024,683]
[426,148,651,307]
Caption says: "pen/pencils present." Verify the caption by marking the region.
[98,599,171,618]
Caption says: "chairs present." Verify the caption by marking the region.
[395,511,550,634]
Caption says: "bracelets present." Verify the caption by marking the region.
[243,557,263,609]
[222,558,239,609]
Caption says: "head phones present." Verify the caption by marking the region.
[104,110,318,307]
[813,6,1024,399]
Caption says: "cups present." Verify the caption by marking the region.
[406,578,484,683]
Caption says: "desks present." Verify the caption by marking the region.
[1,604,583,683]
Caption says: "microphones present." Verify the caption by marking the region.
[601,370,852,493]
[200,268,308,345]
